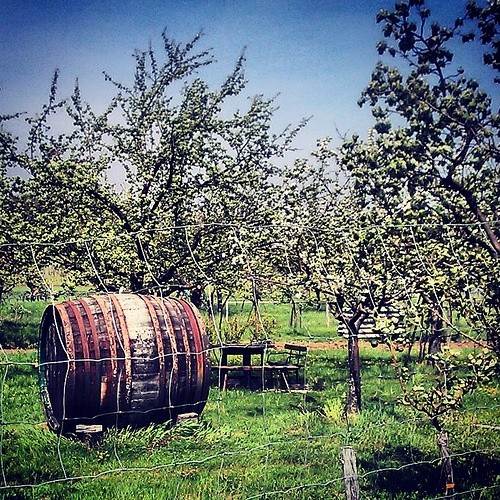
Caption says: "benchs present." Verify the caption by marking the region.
[337,306,407,339]
[210,364,300,392]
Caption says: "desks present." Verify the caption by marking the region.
[220,343,274,387]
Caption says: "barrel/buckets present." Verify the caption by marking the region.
[37,294,211,439]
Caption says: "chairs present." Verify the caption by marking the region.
[265,344,308,389]
